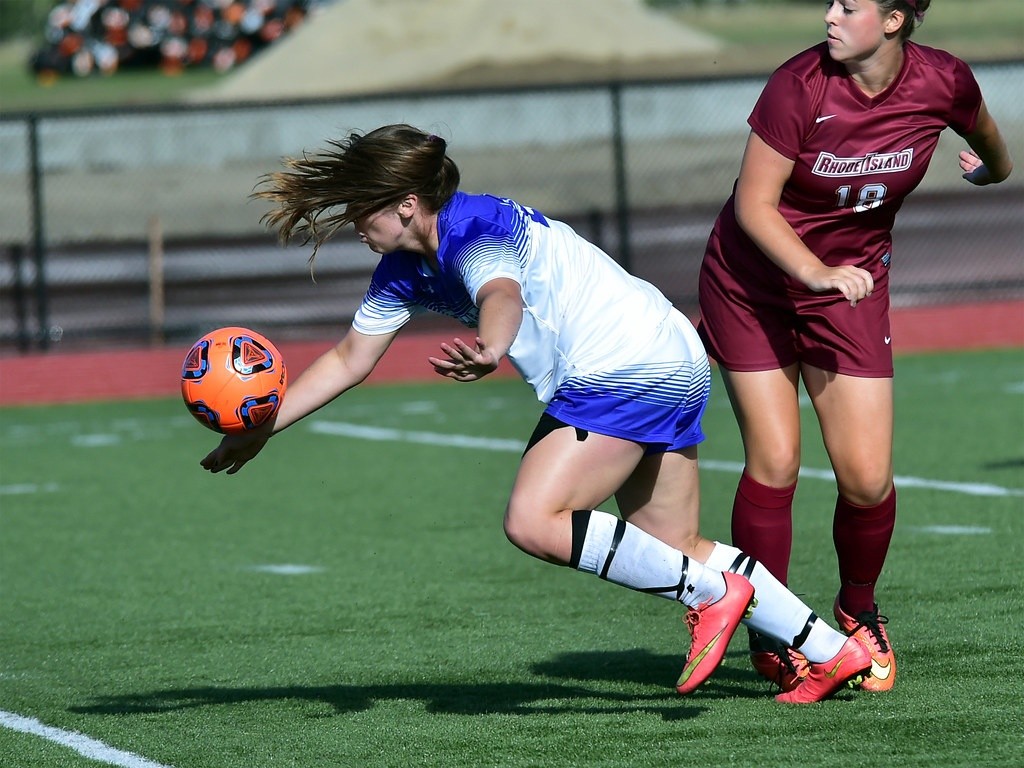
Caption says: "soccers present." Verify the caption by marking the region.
[180,323,288,436]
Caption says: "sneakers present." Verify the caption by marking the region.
[675,571,756,694]
[776,637,872,703]
[749,646,810,692]
[834,592,895,693]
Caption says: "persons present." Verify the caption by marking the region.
[199,126,872,707]
[695,0,1011,692]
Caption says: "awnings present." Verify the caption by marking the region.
[181,0,748,101]
